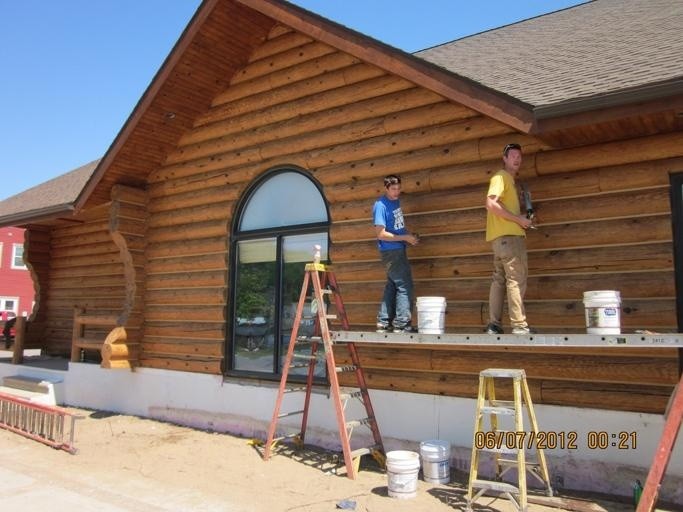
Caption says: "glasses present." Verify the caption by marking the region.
[503,143,521,156]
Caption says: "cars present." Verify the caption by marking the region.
[0,311,18,338]
[233,299,315,351]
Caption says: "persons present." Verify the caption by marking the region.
[372,173,420,334]
[486,143,532,334]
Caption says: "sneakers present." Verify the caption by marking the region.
[487,323,503,334]
[393,321,419,334]
[374,321,394,334]
[511,324,536,335]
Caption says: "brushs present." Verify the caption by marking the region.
[527,226,549,237]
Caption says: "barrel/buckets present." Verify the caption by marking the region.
[384,449,420,499]
[420,439,452,484]
[582,290,621,336]
[415,294,447,335]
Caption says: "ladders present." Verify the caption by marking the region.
[0,392,86,455]
[464,367,552,512]
[263,263,385,480]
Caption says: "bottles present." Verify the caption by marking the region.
[312,245,321,263]
[631,479,643,506]
